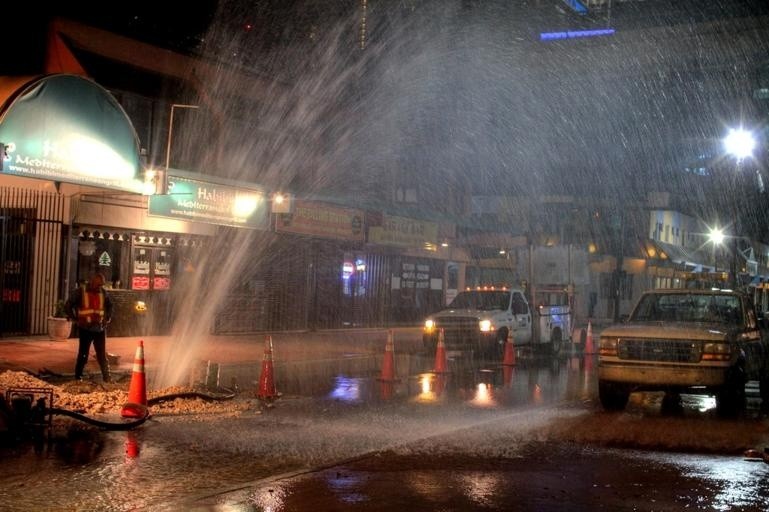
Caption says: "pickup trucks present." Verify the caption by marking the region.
[594,284,769,419]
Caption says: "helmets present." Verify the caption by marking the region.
[91,274,105,288]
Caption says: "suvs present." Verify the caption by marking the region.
[421,285,574,361]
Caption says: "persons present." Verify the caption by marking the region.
[62,272,116,382]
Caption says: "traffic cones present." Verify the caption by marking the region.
[255,331,279,400]
[117,337,153,420]
[374,328,402,382]
[578,319,598,356]
[427,327,451,376]
[499,324,519,366]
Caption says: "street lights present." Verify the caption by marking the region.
[708,226,726,273]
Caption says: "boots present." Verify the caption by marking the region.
[75,364,85,380]
[102,364,116,384]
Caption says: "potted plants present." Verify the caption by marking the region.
[47,298,74,340]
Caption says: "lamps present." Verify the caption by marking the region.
[434,236,448,247]
[545,236,554,246]
[79,238,96,256]
[496,245,506,255]
[3,142,17,162]
